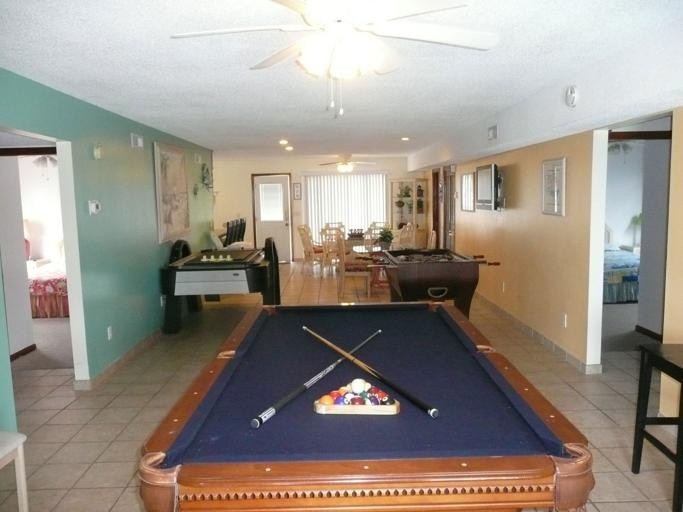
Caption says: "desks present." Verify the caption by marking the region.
[630,343,683,512]
[0,429,29,512]
[159,237,280,334]
[354,249,500,319]
[135,300,595,512]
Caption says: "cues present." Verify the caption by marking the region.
[301,324,437,417]
[250,327,383,430]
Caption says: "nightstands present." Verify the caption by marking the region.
[623,244,640,256]
[27,256,47,270]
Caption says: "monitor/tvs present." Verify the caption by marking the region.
[475,163,502,211]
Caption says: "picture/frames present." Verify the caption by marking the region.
[293,182,303,201]
[458,172,476,212]
[151,140,191,246]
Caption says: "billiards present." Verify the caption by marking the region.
[314,376,401,414]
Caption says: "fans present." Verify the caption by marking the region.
[315,153,377,173]
[162,0,498,117]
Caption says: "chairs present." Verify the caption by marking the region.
[294,221,437,301]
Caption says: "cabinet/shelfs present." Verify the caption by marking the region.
[387,176,428,249]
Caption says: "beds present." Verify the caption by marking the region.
[602,223,642,304]
[27,239,69,321]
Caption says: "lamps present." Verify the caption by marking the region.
[294,30,392,115]
